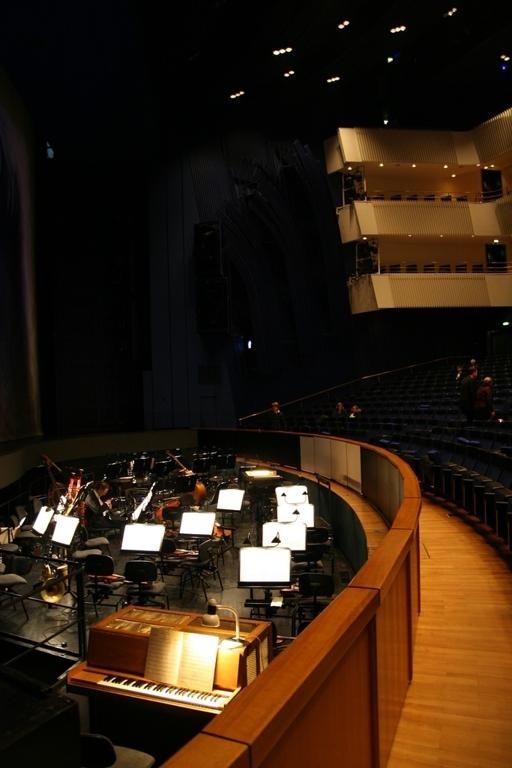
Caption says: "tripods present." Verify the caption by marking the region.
[63,547,78,601]
[195,537,210,602]
[218,512,240,556]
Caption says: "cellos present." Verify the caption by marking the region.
[168,450,207,507]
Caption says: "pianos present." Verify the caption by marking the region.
[68,605,272,743]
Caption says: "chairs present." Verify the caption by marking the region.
[358,192,470,202]
[0,448,356,643]
[376,262,485,273]
[241,361,512,559]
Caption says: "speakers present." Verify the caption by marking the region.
[192,220,233,335]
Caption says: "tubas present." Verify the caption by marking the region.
[39,563,65,604]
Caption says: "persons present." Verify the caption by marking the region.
[84,481,132,554]
[454,367,464,389]
[462,366,479,426]
[332,401,347,418]
[468,359,477,367]
[475,376,495,421]
[347,405,362,418]
[270,401,284,416]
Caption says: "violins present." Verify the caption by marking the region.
[171,548,199,558]
[88,573,147,584]
